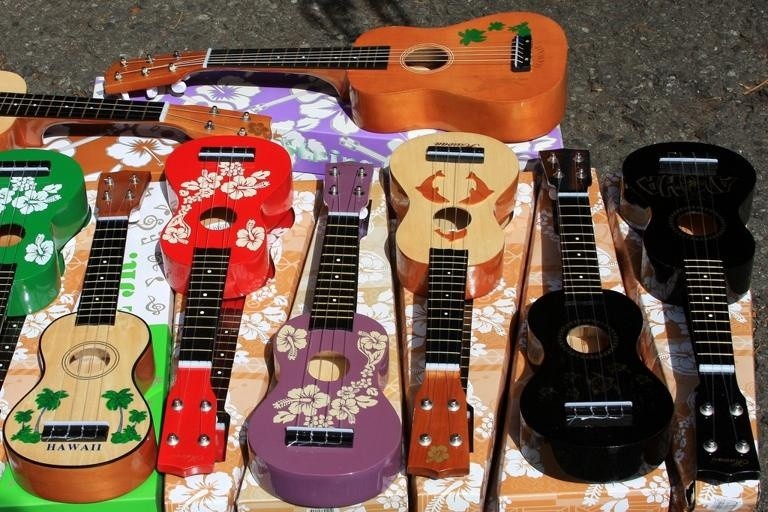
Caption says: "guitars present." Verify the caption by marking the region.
[1,148,92,413]
[156,135,293,477]
[0,70,273,151]
[2,169,158,505]
[617,142,761,485]
[388,131,519,479]
[104,11,569,143]
[520,149,677,484]
[246,161,404,508]
